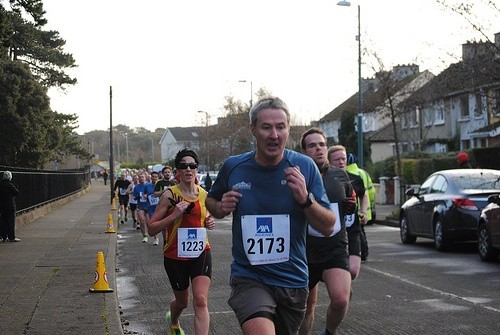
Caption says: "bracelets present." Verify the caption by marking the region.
[361,211,365,214]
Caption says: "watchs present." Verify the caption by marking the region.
[296,192,314,209]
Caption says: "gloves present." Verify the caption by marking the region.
[113,193,115,198]
[168,193,195,214]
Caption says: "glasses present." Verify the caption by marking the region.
[179,162,199,170]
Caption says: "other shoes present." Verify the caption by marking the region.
[125,214,128,222]
[142,236,148,243]
[133,218,140,230]
[152,238,158,245]
[121,219,124,224]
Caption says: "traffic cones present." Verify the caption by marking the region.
[104,213,116,233]
[110,198,117,210]
[88,249,114,293]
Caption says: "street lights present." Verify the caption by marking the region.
[198,110,210,173]
[239,79,253,108]
[336,0,365,168]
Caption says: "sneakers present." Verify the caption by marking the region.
[165,311,185,335]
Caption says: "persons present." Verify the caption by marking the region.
[203,97,336,335]
[285,127,352,335]
[343,152,375,262]
[89,165,216,244]
[455,152,473,169]
[147,148,217,335]
[0,170,21,242]
[325,146,370,302]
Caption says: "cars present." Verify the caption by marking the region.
[477,194,500,262]
[398,167,500,251]
[198,174,217,188]
